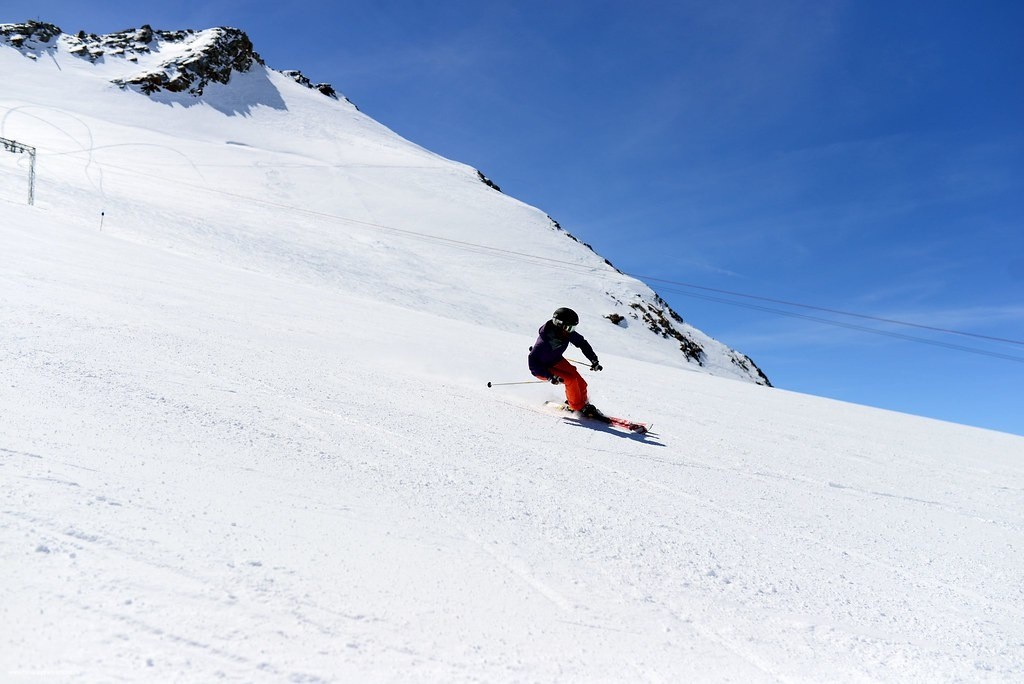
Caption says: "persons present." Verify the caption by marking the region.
[528,307,599,414]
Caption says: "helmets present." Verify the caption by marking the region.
[552,308,579,325]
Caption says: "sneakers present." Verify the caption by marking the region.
[567,402,596,418]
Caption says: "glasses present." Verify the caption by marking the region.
[563,325,576,332]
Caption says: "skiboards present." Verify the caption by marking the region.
[543,399,655,435]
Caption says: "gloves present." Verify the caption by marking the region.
[547,374,561,385]
[590,356,599,371]
